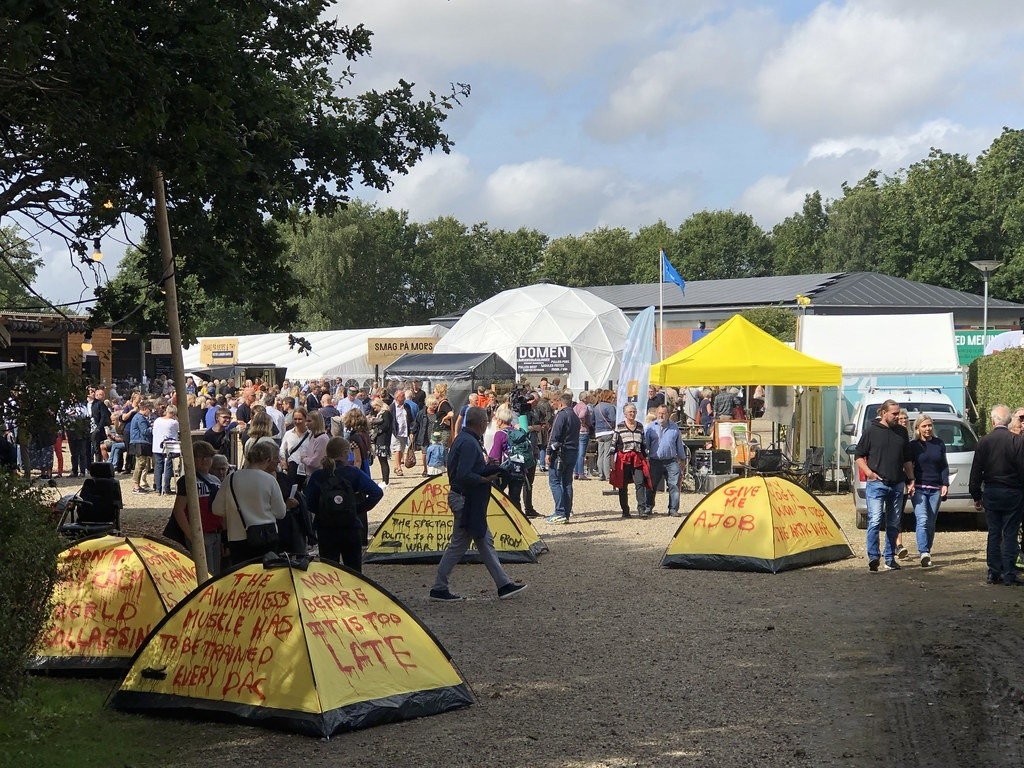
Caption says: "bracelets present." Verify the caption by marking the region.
[908,478,916,481]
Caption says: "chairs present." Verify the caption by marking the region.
[939,430,964,453]
[731,430,761,477]
[744,445,824,493]
[61,462,123,536]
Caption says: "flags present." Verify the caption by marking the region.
[662,252,685,297]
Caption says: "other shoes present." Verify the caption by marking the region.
[525,509,545,519]
[66,471,85,479]
[157,490,176,497]
[394,467,403,476]
[122,470,132,475]
[668,508,682,517]
[422,470,427,477]
[987,571,1024,586]
[622,506,652,519]
[573,474,591,480]
[378,481,390,489]
[39,473,63,480]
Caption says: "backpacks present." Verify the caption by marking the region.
[319,467,357,517]
[498,427,536,491]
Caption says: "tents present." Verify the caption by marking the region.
[180,324,450,388]
[658,472,856,574]
[102,551,481,740]
[23,529,214,669]
[361,471,550,564]
[383,352,517,394]
[433,283,634,403]
[650,314,842,493]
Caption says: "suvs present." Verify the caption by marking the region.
[845,410,987,529]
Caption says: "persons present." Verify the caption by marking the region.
[647,385,766,436]
[429,407,527,600]
[853,400,949,571]
[609,404,686,520]
[968,403,1024,586]
[0,374,616,569]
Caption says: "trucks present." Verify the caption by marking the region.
[843,385,957,480]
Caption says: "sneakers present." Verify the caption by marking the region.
[132,483,150,494]
[430,589,462,601]
[895,544,908,559]
[546,513,569,525]
[868,559,902,571]
[498,582,528,600]
[921,552,932,567]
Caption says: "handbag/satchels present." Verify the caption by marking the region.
[405,438,417,468]
[246,523,281,546]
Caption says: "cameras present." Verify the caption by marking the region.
[550,442,567,470]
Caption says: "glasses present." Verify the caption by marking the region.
[219,415,233,421]
